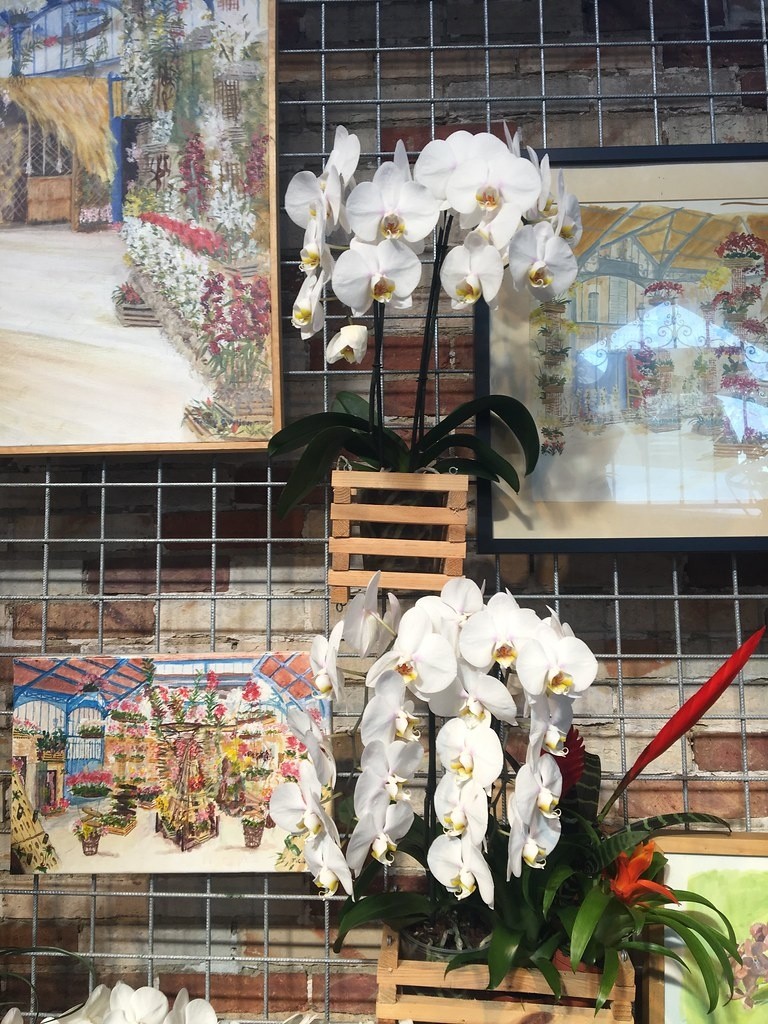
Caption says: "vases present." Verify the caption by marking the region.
[550,952,601,1008]
[359,488,444,598]
[398,903,497,1023]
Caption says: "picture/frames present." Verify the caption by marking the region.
[0,0,284,455]
[467,142,768,554]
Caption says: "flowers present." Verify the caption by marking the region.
[268,123,585,519]
[265,571,745,1016]
[0,946,241,1024]
